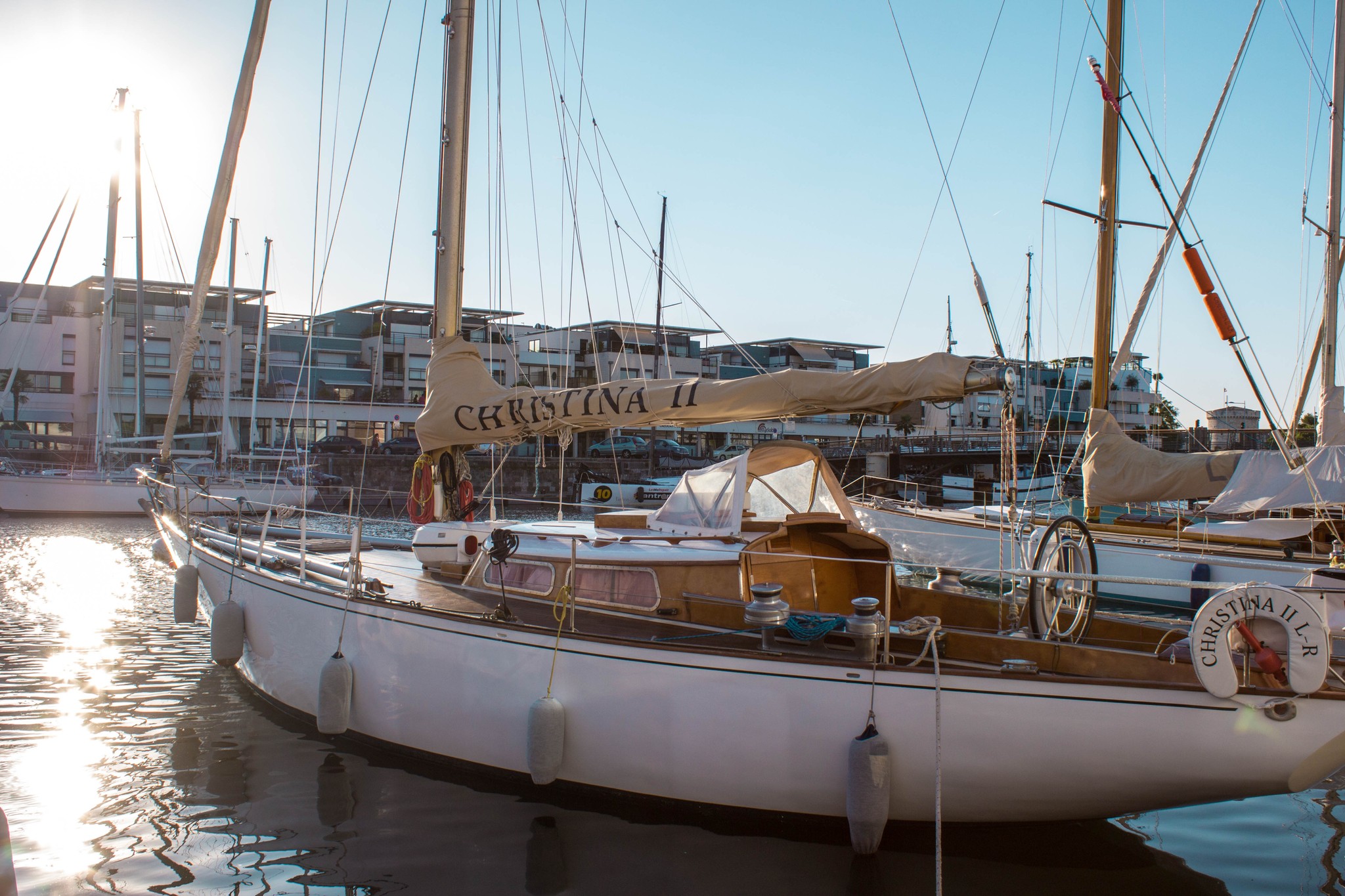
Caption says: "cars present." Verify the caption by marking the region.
[642,439,689,459]
[463,444,497,456]
[821,446,859,457]
[298,435,365,454]
[707,444,747,461]
[587,436,648,459]
[370,436,422,456]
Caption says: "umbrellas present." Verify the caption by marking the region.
[275,380,301,399]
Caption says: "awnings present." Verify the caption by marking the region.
[613,327,662,346]
[319,378,373,386]
[787,343,836,363]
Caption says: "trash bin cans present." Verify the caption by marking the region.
[259,443,266,455]
[544,444,555,457]
[553,444,560,457]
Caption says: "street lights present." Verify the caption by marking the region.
[1227,401,1245,429]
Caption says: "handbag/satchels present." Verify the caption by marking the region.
[377,442,382,447]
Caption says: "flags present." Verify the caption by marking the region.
[1224,388,1227,392]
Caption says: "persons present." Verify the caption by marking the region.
[412,394,425,408]
[591,438,597,446]
[371,433,382,454]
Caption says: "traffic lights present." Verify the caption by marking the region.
[1240,422,1245,429]
[1196,419,1200,427]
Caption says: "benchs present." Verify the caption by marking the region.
[889,626,946,658]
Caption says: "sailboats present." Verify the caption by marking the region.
[126,0,1344,828]
[572,191,684,507]
[897,245,1065,506]
[836,0,1344,661]
[0,88,344,517]
